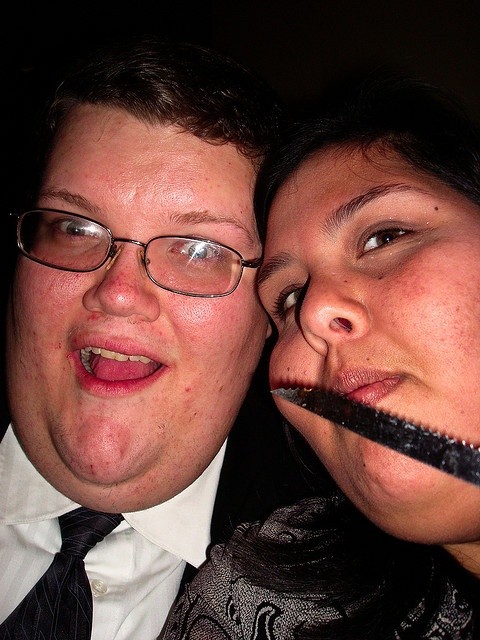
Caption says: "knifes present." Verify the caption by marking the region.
[268,378,480,489]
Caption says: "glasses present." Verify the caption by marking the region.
[10,208,262,298]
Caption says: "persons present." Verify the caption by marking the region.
[160,84,480,634]
[0,20,286,636]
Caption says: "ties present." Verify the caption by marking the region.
[0,506,125,640]
